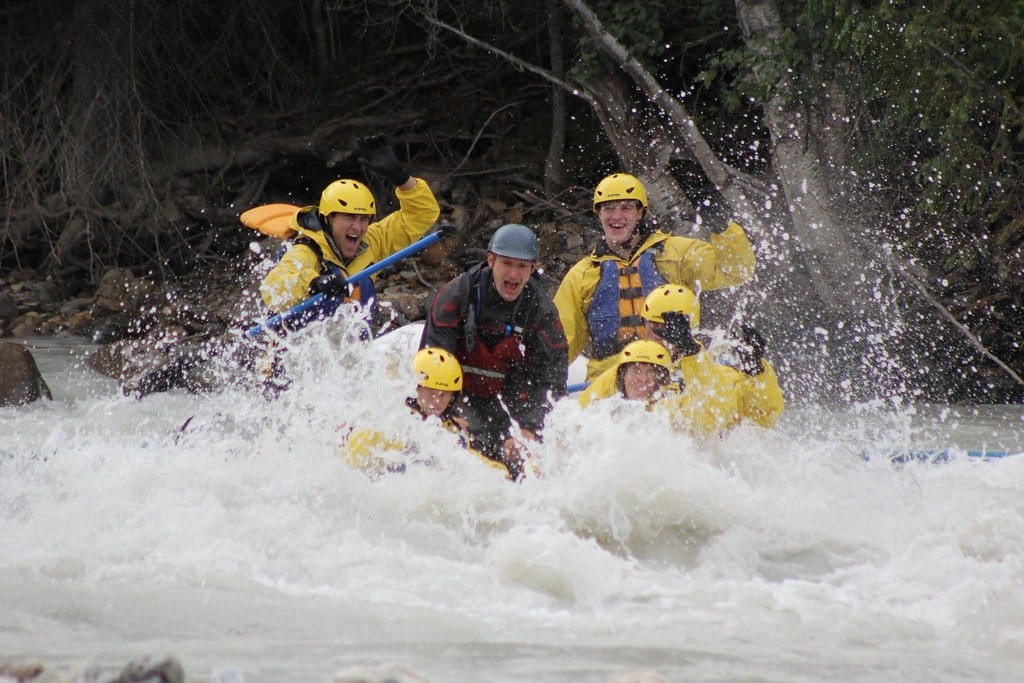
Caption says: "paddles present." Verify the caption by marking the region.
[568,380,591,392]
[117,219,461,400]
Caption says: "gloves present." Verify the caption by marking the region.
[310,275,353,298]
[732,324,766,376]
[666,157,733,234]
[355,133,409,186]
[654,311,700,355]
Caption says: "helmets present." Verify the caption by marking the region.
[615,340,671,391]
[318,179,377,218]
[486,224,538,260]
[593,173,648,213]
[641,284,700,326]
[411,348,463,391]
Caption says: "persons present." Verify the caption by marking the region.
[258,136,442,383]
[345,347,512,481]
[550,172,758,382]
[613,340,675,412]
[417,224,568,482]
[578,282,784,435]
